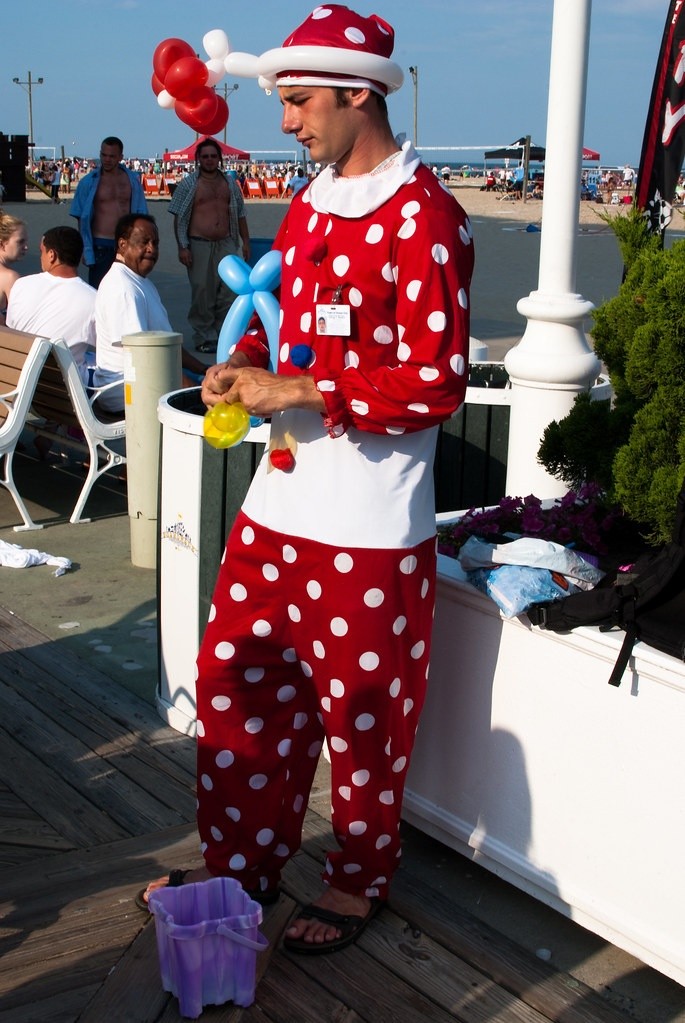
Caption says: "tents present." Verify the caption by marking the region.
[582,148,600,169]
[163,135,250,178]
[483,138,545,186]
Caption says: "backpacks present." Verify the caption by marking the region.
[527,477,685,686]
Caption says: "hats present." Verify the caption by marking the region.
[275,2,394,97]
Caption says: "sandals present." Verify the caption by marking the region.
[284,885,383,954]
[135,865,276,914]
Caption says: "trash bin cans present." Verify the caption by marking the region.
[156,385,271,739]
[433,361,614,512]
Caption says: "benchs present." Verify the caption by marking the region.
[0,327,53,532]
[22,330,127,526]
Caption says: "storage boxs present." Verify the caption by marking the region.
[146,876,264,1018]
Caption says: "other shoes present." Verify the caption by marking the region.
[82,454,108,470]
[34,435,52,461]
[69,448,86,463]
[118,465,127,481]
[196,337,217,354]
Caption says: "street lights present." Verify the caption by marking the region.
[212,81,239,146]
[408,65,419,148]
[12,70,44,174]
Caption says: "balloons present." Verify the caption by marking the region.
[152,28,405,134]
[216,249,282,428]
[203,401,248,450]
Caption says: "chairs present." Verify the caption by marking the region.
[493,185,518,201]
[142,172,292,199]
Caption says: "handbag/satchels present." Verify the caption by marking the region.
[459,529,604,618]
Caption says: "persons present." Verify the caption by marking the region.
[0,210,29,327]
[26,155,95,203]
[167,139,251,353]
[318,317,326,333]
[122,157,191,181]
[675,176,685,204]
[487,167,513,188]
[459,164,468,179]
[223,160,321,194]
[426,163,451,183]
[69,137,149,291]
[136,4,475,956]
[93,213,212,414]
[6,226,98,395]
[512,161,524,200]
[606,165,635,205]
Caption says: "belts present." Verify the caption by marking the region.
[188,235,217,243]
